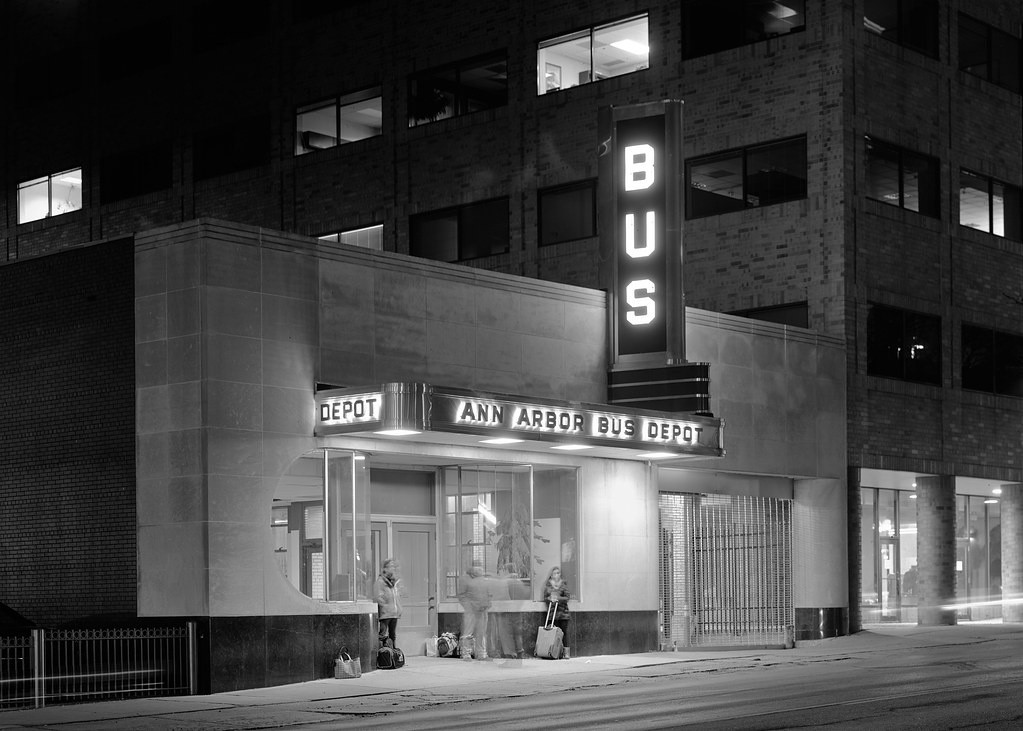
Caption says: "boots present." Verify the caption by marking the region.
[562,646,570,659]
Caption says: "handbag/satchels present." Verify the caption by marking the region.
[332,647,362,679]
[438,631,461,657]
[425,635,440,657]
[374,646,405,669]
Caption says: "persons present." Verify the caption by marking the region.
[453,560,530,664]
[543,567,572,660]
[372,559,404,649]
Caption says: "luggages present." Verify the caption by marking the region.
[532,599,565,661]
